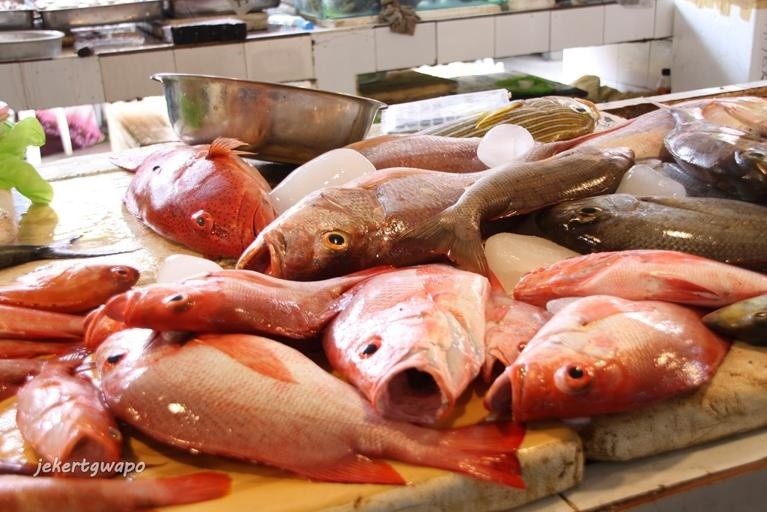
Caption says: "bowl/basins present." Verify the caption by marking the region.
[147,72,391,168]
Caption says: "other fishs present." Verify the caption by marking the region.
[0,95,767,512]
